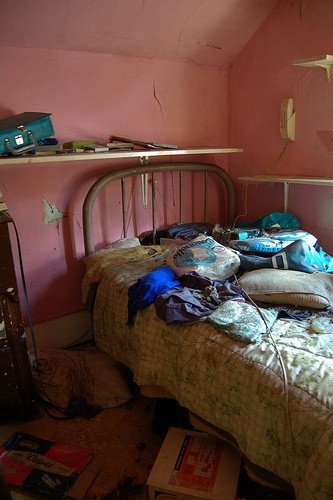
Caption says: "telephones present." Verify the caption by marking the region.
[278,97,297,141]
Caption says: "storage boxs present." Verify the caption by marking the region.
[146,427,241,500]
[0,112,56,153]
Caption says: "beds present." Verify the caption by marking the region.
[82,164,333,500]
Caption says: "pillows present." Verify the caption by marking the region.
[166,235,241,283]
[232,268,329,309]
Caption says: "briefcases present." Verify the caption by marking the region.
[0,112,56,155]
